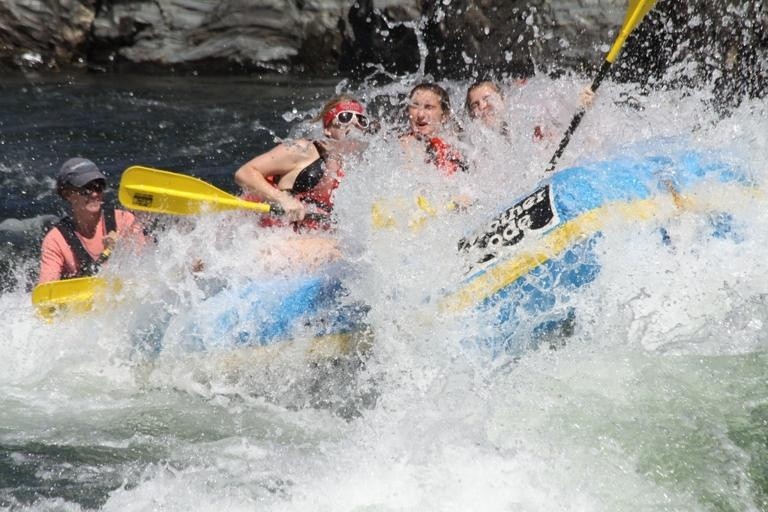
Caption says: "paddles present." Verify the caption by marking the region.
[33,276,119,320]
[119,165,338,221]
[544,1,656,174]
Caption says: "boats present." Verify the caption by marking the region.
[22,139,767,398]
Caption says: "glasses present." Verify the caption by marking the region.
[82,183,106,192]
[331,110,370,129]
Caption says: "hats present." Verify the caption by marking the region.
[58,158,108,192]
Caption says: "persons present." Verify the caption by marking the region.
[38,156,145,286]
[232,97,371,234]
[395,80,468,178]
[465,81,594,147]
